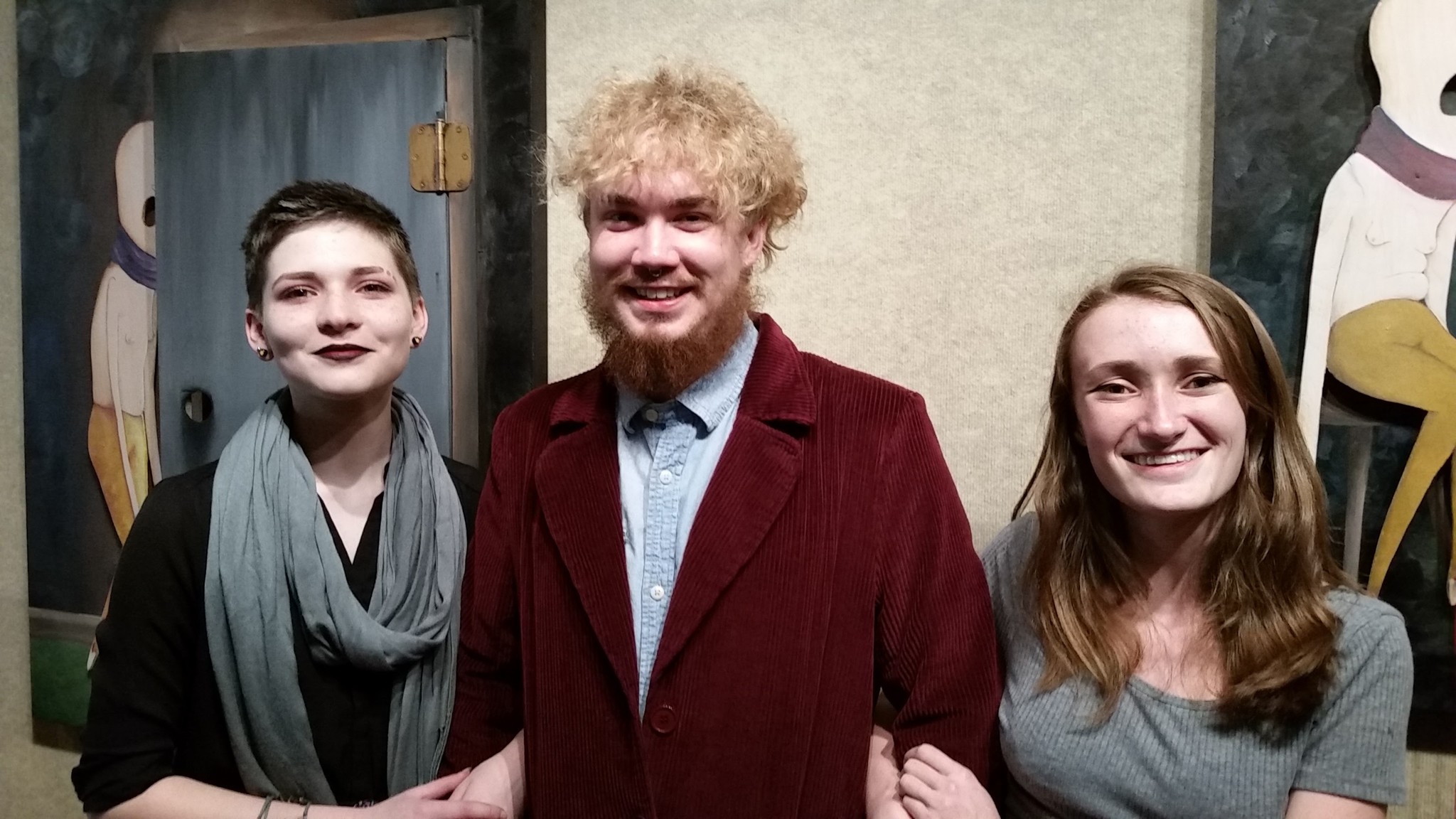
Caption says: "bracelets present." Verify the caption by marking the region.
[303,801,310,818]
[258,795,273,818]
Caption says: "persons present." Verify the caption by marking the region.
[864,268,1415,818]
[435,70,1004,819]
[69,183,525,819]
